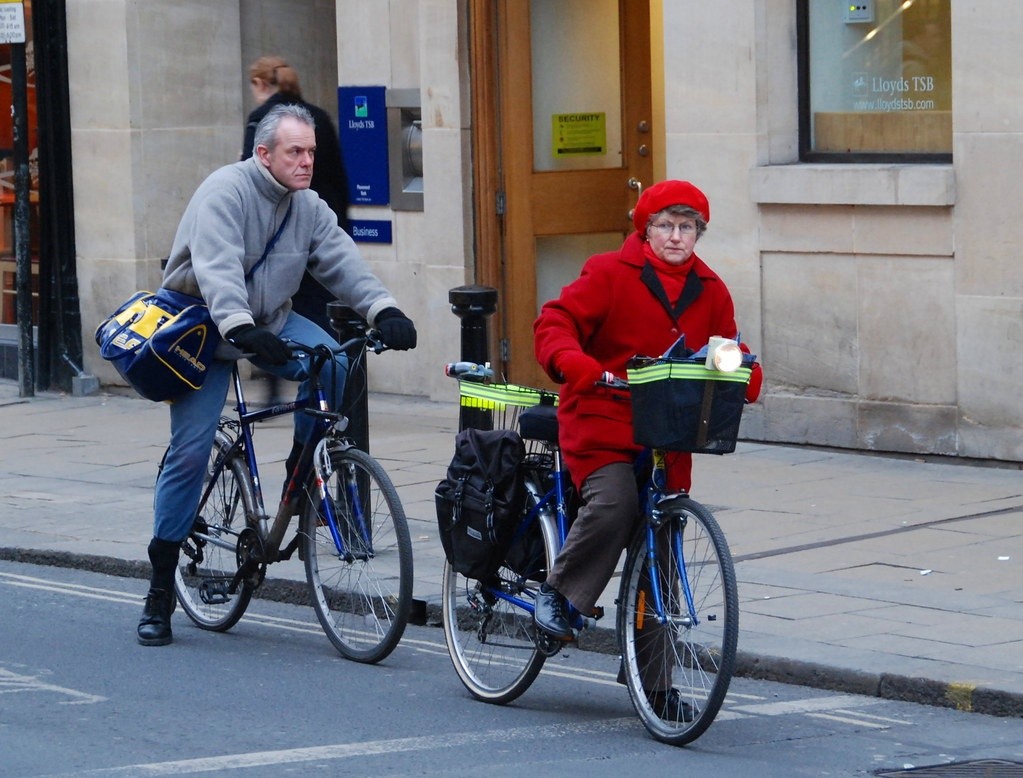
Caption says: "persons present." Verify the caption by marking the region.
[137,105,416,647]
[240,56,351,422]
[532,180,762,723]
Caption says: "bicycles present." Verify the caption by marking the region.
[153,320,418,664]
[438,358,761,748]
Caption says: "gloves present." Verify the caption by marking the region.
[225,323,293,364]
[373,307,416,350]
[553,349,602,394]
[744,363,763,404]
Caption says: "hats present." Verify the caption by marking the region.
[633,180,709,242]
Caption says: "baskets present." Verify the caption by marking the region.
[459,378,559,464]
[625,363,752,456]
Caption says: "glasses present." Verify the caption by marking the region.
[650,219,698,234]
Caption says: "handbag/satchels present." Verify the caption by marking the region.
[433,428,522,578]
[95,291,223,402]
[508,447,580,582]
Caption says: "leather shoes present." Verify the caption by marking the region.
[137,586,177,645]
[279,487,351,525]
[534,584,572,642]
[644,686,699,723]
